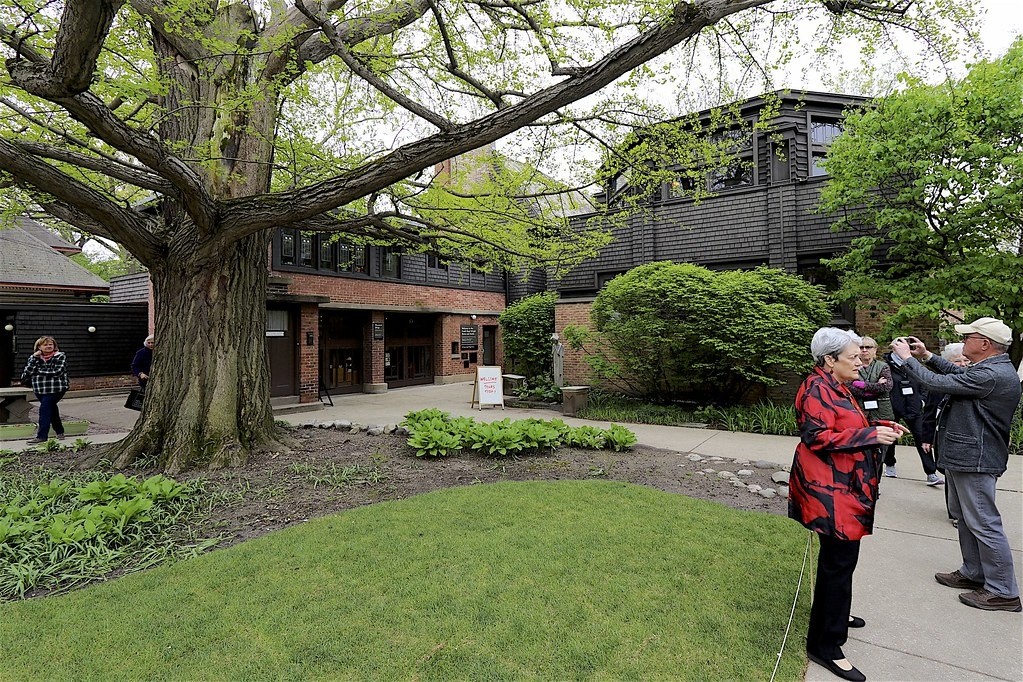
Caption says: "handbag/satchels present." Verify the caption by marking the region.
[124,376,149,411]
[20,373,32,388]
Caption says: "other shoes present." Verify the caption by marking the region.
[26,437,44,445]
[56,433,65,440]
[848,614,866,628]
[807,651,866,682]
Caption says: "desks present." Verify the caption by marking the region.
[0,387,33,426]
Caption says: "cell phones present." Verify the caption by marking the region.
[898,338,916,350]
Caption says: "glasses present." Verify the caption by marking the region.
[859,344,876,349]
[963,335,987,341]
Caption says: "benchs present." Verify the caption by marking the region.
[560,386,590,417]
[502,374,526,394]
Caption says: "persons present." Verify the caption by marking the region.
[20,337,69,444]
[843,336,971,486]
[787,327,910,682]
[550,333,564,386]
[132,334,155,391]
[890,317,1022,612]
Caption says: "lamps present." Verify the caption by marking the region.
[471,314,477,319]
[5,323,13,331]
[88,324,96,333]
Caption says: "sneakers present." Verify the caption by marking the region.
[934,569,985,590]
[926,473,945,486]
[885,463,898,478]
[958,588,1022,612]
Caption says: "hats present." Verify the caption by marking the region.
[954,317,1014,346]
